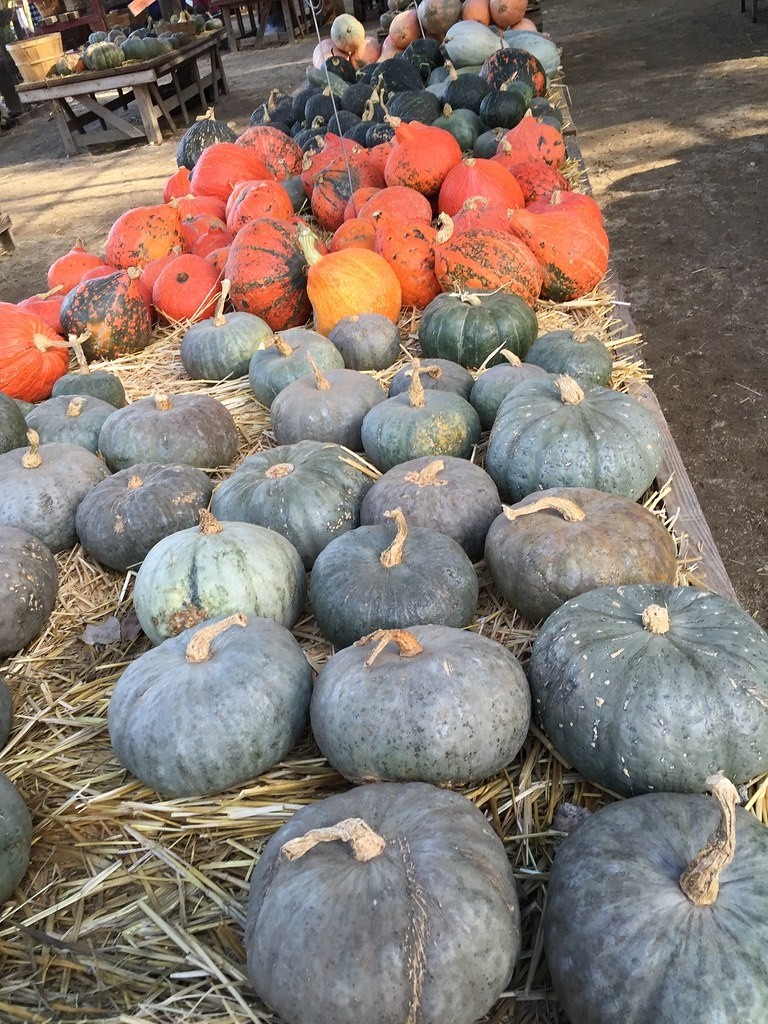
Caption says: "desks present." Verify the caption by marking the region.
[15,25,233,156]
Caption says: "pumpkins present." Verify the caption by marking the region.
[2,1,766,1022]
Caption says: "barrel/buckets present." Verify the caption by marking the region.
[33,0,61,18]
[153,20,196,46]
[5,32,64,84]
